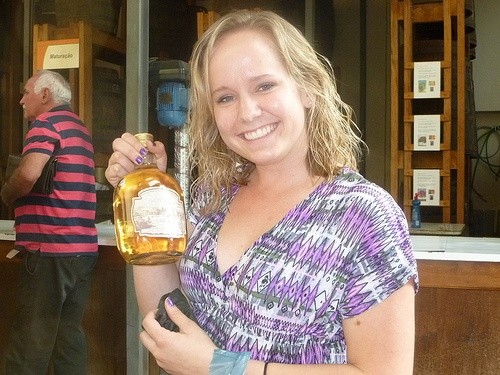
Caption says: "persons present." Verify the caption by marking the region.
[106,9,419,375]
[0,69,98,375]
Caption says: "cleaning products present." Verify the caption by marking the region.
[412,193,421,228]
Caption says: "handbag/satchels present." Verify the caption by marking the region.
[5,154,57,195]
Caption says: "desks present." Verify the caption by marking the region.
[408,222,466,236]
[0,227,500,375]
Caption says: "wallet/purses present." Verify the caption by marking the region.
[158,289,202,335]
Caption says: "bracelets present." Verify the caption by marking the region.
[263,362,268,375]
